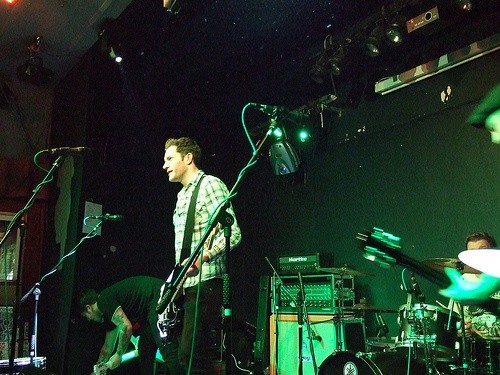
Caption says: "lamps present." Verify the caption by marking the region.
[97,18,125,62]
[265,127,301,177]
[455,1,474,10]
[363,30,381,56]
[387,11,402,43]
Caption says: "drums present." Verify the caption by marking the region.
[317,349,426,375]
[449,334,500,375]
[392,303,463,363]
[366,336,399,353]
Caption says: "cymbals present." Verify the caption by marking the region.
[420,258,483,274]
[316,267,375,277]
[322,304,398,313]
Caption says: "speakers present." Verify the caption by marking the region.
[269,315,341,375]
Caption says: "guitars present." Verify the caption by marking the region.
[355,224,500,319]
[93,336,166,375]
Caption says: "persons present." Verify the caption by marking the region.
[448,86,500,343]
[79,276,186,375]
[162,138,242,375]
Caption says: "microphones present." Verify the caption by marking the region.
[411,272,425,302]
[42,146,90,155]
[249,103,310,127]
[89,214,123,223]
